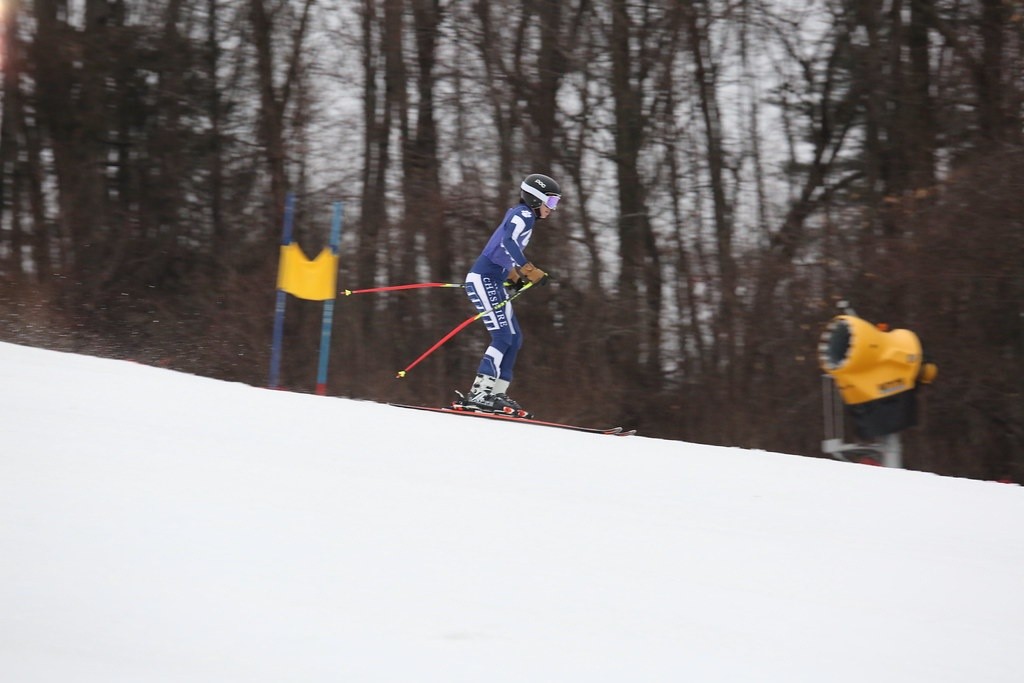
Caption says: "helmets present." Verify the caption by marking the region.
[520,173,562,208]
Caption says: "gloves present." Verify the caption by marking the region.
[512,277,524,292]
[540,272,553,286]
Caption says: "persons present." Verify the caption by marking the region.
[470,173,562,419]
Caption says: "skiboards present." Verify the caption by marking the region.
[389,402,638,437]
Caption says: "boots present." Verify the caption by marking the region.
[465,372,512,410]
[485,392,523,410]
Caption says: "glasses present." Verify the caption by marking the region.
[544,195,560,210]
